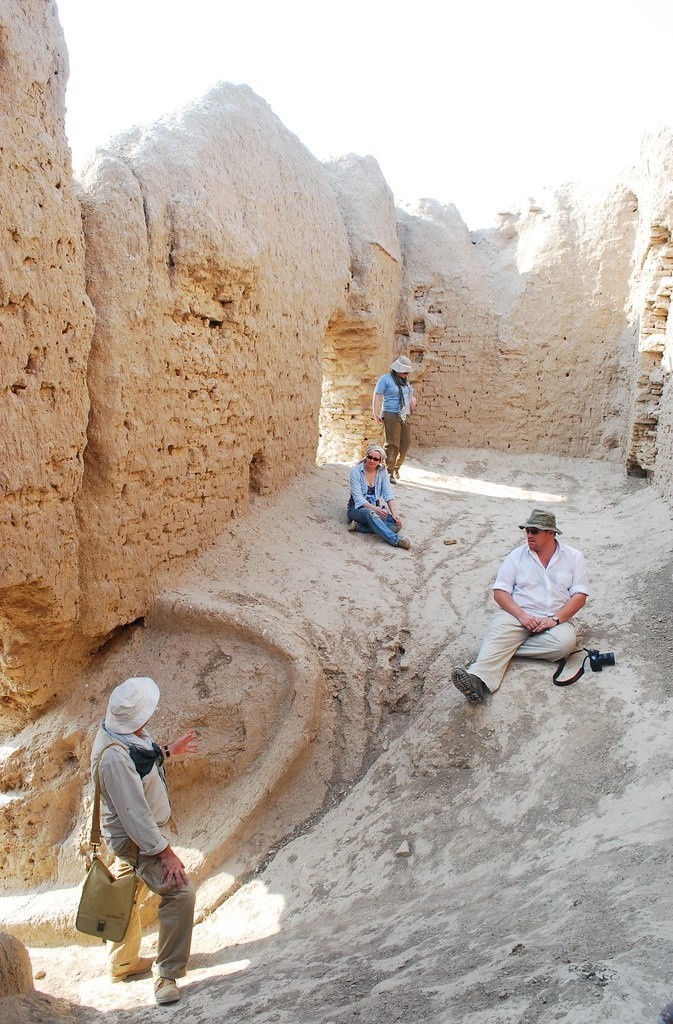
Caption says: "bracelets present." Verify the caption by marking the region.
[163,744,171,758]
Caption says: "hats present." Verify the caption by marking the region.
[390,356,414,373]
[519,509,562,535]
[105,677,160,734]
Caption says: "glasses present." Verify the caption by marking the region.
[526,528,548,534]
[367,455,381,461]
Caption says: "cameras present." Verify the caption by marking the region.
[588,649,615,672]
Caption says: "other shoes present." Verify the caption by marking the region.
[394,470,399,479]
[390,476,396,484]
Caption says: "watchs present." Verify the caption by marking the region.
[548,615,560,625]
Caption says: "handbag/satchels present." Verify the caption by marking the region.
[76,856,136,943]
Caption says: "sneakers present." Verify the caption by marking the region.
[452,668,485,703]
[153,972,181,1004]
[398,536,410,549]
[110,956,157,982]
[348,520,356,531]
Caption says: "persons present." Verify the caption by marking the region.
[347,446,411,549]
[452,509,591,704]
[373,355,417,484]
[91,677,199,1004]
